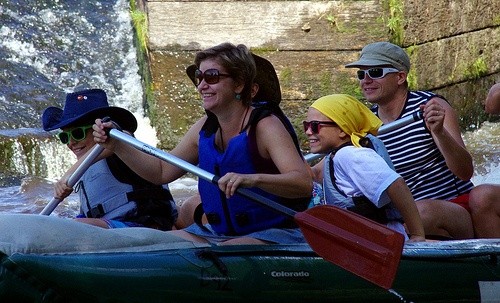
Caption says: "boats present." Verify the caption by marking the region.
[0,210,496,303]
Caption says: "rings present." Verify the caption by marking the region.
[229,179,235,184]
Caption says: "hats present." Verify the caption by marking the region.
[345,42,411,74]
[42,89,137,134]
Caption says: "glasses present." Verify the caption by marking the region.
[194,69,231,84]
[57,125,92,145]
[358,67,399,80]
[302,120,336,133]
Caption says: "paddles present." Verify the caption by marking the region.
[38,120,123,216]
[303,108,425,164]
[100,115,406,292]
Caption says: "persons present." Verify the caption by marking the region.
[309,42,474,240]
[42,88,176,231]
[302,93,425,241]
[92,42,313,246]
[468,82,500,240]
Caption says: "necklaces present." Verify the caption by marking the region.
[219,104,249,154]
